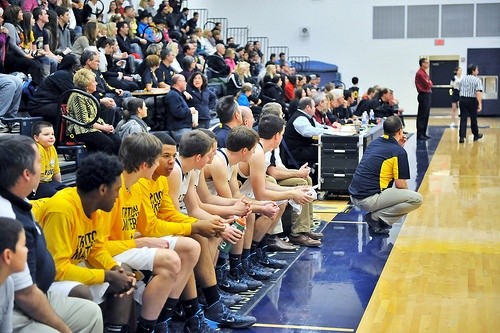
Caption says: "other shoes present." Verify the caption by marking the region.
[450,123,458,127]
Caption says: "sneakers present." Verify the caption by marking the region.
[152,230,324,333]
[0,120,20,133]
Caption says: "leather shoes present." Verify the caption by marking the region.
[417,135,431,140]
[363,211,392,235]
[474,134,483,140]
[459,138,465,143]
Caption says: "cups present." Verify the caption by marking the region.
[146,84,151,92]
[192,111,198,124]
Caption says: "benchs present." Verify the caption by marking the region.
[0,83,224,185]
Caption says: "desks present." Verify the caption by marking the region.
[319,118,386,193]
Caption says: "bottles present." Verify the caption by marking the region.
[218,218,246,254]
[360,111,368,129]
[370,109,374,120]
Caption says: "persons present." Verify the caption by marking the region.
[0,0,405,333]
[450,67,464,126]
[415,57,433,139]
[0,135,103,333]
[0,217,28,333]
[35,152,138,333]
[459,64,483,144]
[102,131,201,333]
[348,115,423,234]
